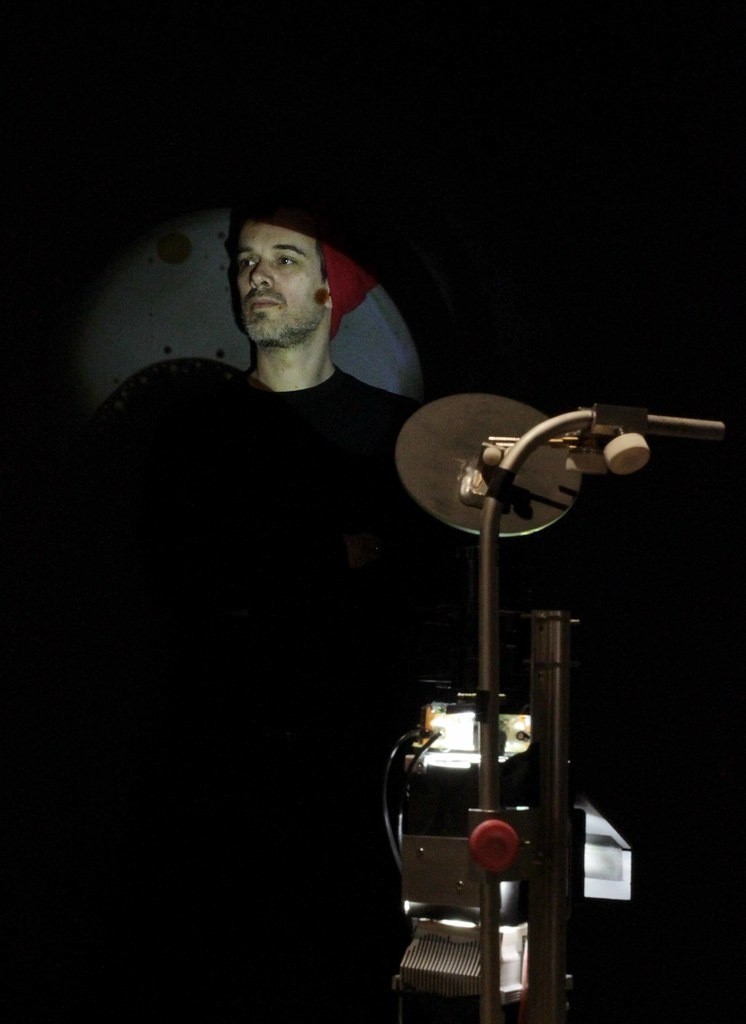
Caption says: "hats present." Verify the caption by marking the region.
[317,197,383,341]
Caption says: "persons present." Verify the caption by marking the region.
[88,168,447,919]
